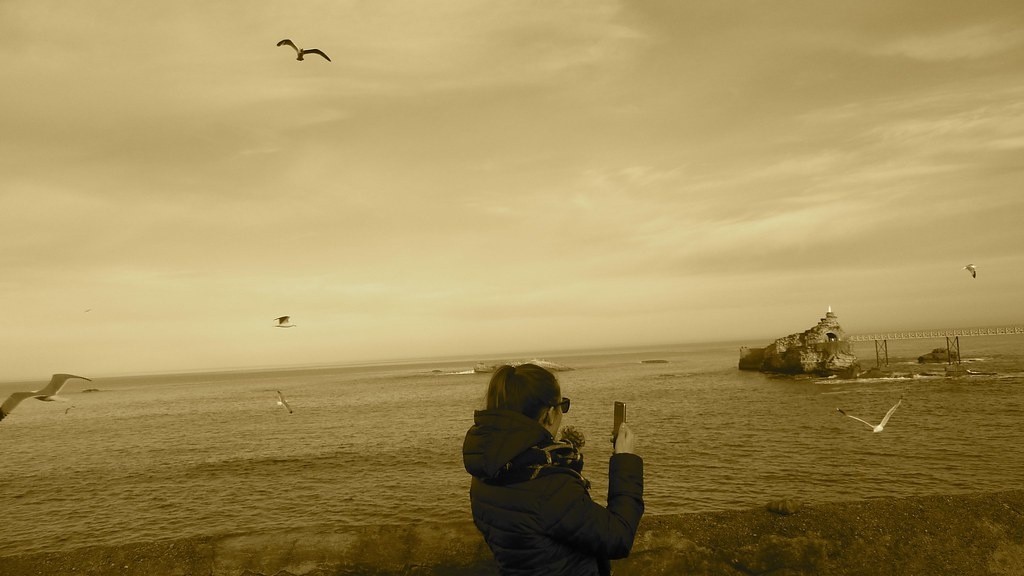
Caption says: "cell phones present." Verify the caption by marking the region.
[613,401,626,441]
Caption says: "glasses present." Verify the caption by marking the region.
[543,397,570,424]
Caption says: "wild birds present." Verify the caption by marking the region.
[277,390,293,415]
[273,315,297,329]
[837,396,903,434]
[962,263,978,279]
[0,373,92,422]
[275,38,332,66]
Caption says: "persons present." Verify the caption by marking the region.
[462,364,645,576]
